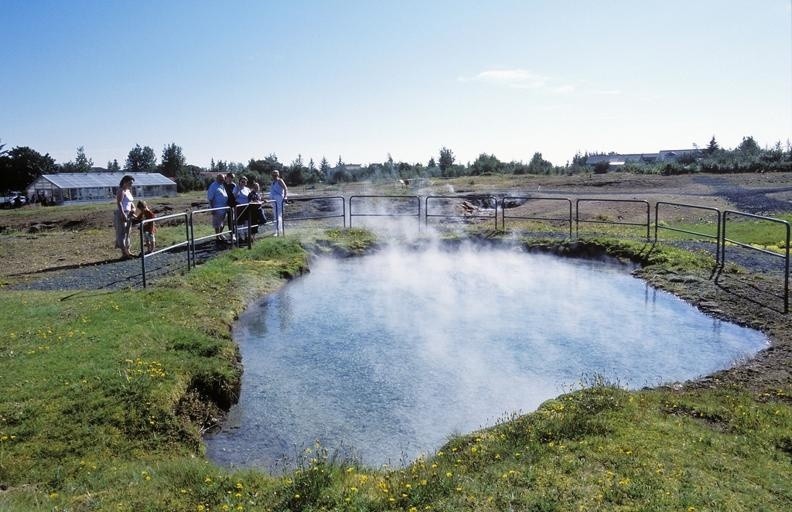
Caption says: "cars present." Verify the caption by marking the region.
[0,190,26,210]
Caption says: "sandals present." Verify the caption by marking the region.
[123,254,136,259]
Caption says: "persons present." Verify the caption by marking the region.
[134,200,158,255]
[231,176,251,242]
[206,173,229,243]
[252,178,266,197]
[225,173,236,240]
[247,182,269,244]
[268,170,288,238]
[112,175,140,261]
